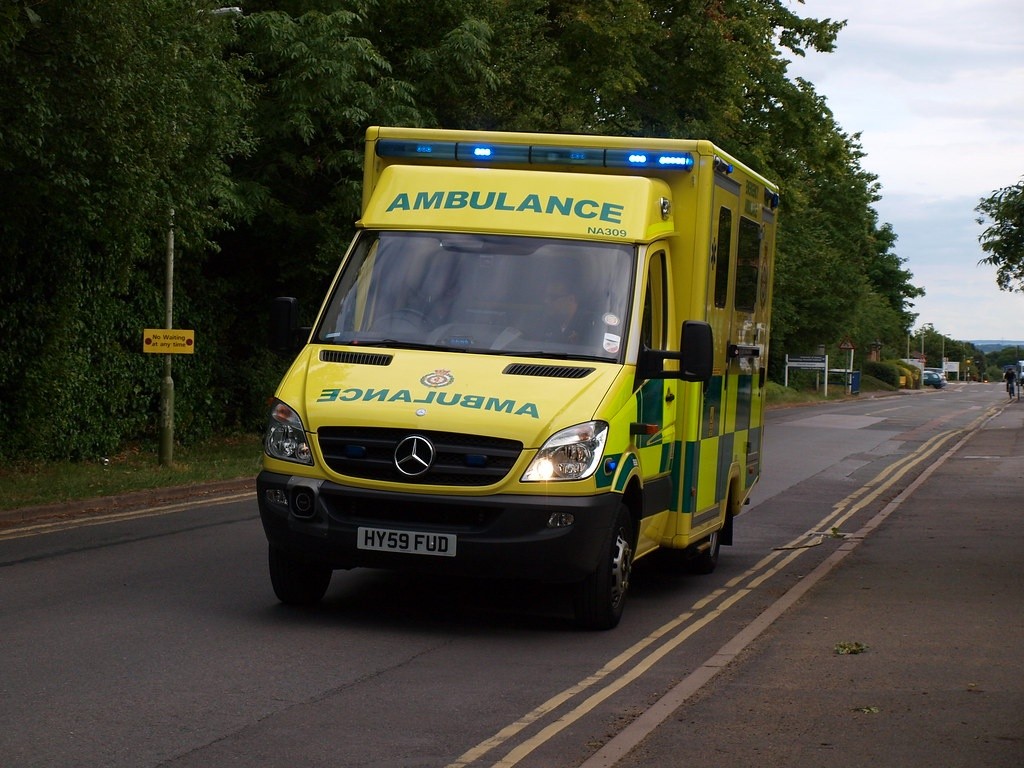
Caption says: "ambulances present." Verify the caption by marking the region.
[253,123,784,630]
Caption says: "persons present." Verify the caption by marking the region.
[1004,368,1016,396]
[525,277,608,347]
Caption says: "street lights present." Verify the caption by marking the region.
[941,334,951,371]
[920,322,932,363]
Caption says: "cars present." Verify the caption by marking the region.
[923,370,946,389]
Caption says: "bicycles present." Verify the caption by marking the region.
[1004,378,1017,399]
